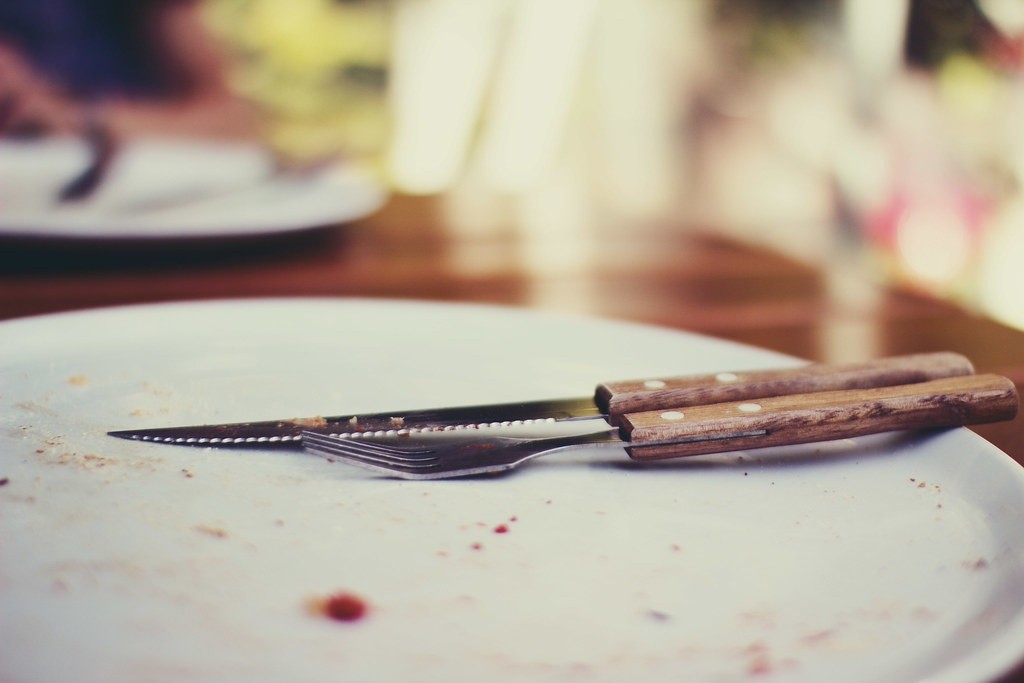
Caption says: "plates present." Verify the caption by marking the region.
[0,299,1024,682]
[0,130,390,253]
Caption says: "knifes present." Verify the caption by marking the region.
[106,349,978,444]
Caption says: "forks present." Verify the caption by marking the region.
[299,373,1020,481]
